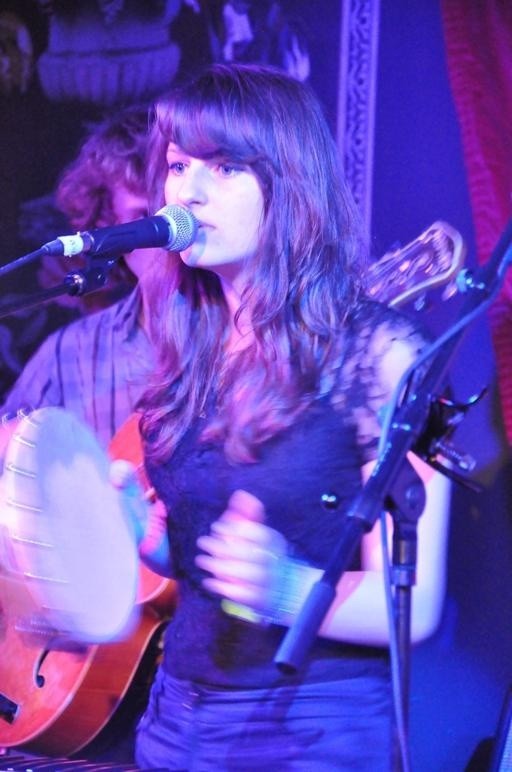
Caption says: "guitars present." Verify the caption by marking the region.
[1,221,458,758]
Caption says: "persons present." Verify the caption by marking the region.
[0,104,187,453]
[117,62,458,772]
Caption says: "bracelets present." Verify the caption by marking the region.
[264,558,310,624]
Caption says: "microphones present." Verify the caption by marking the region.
[43,204,199,259]
